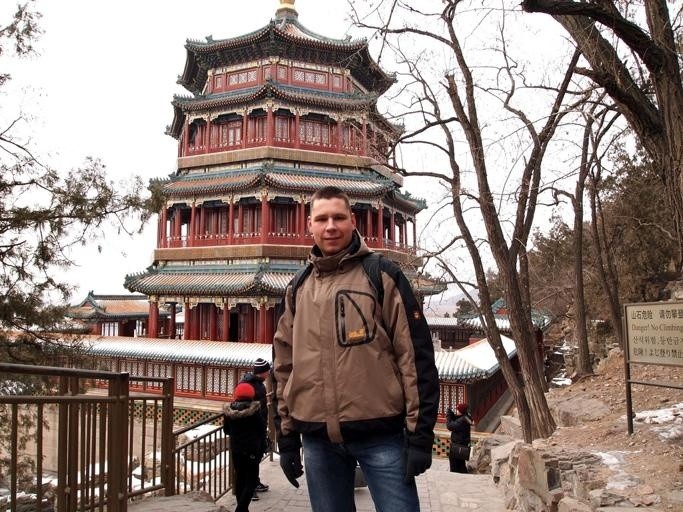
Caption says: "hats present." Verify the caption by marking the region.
[252,358,271,376]
[457,403,468,414]
[235,383,255,399]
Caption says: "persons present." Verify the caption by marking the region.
[240,357,271,501]
[269,184,442,511]
[220,380,266,512]
[443,402,476,475]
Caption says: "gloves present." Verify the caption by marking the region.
[446,408,452,416]
[401,439,431,481]
[280,450,304,488]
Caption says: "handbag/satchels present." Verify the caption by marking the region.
[450,444,470,460]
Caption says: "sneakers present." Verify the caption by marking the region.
[250,494,260,500]
[255,482,269,492]
